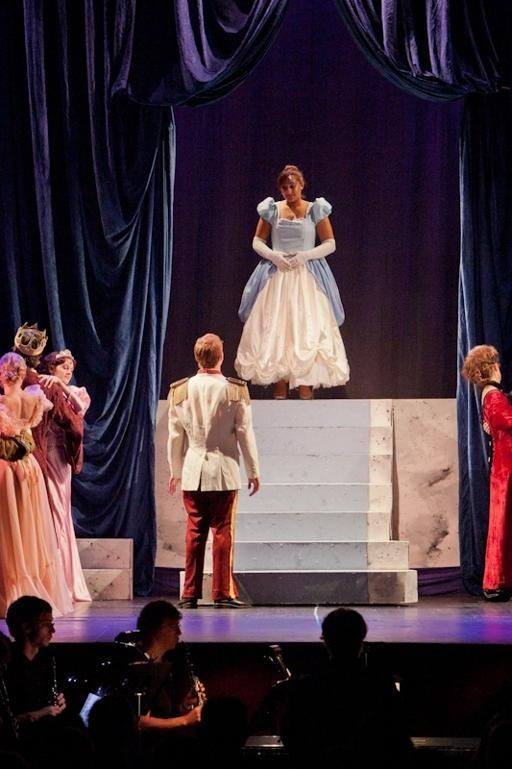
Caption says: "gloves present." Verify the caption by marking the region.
[252,236,336,273]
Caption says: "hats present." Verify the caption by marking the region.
[14,322,49,357]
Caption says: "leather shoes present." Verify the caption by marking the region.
[176,598,251,609]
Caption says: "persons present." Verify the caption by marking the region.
[165,333,261,608]
[457,342,510,602]
[265,609,417,766]
[2,596,66,766]
[119,599,207,769]
[0,323,92,617]
[233,162,351,402]
